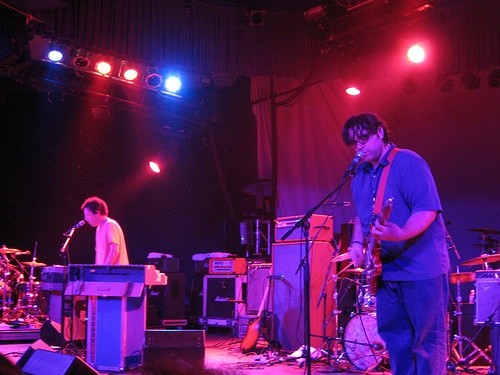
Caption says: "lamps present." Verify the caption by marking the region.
[144,66,163,88]
[397,66,500,95]
[345,79,361,96]
[149,157,171,173]
[72,48,90,69]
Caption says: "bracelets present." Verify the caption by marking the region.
[350,239,364,246]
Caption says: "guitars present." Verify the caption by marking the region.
[367,197,394,297]
[239,266,273,352]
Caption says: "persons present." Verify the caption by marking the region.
[80,196,130,266]
[341,114,449,375]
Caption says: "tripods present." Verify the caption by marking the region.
[270,203,336,374]
[449,276,492,371]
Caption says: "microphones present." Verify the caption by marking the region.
[373,343,384,351]
[66,220,85,234]
[331,201,351,206]
[329,239,340,254]
[344,150,364,177]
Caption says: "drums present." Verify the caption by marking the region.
[341,311,393,371]
[359,291,382,311]
[449,272,476,305]
[17,278,44,306]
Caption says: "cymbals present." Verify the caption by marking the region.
[20,261,49,267]
[460,253,500,265]
[343,266,368,276]
[0,248,30,256]
[331,247,365,264]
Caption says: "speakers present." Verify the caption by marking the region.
[141,330,205,374]
[246,214,338,353]
[0,320,99,375]
[475,270,500,324]
[156,272,185,319]
[203,275,247,321]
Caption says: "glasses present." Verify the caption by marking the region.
[346,129,372,146]
